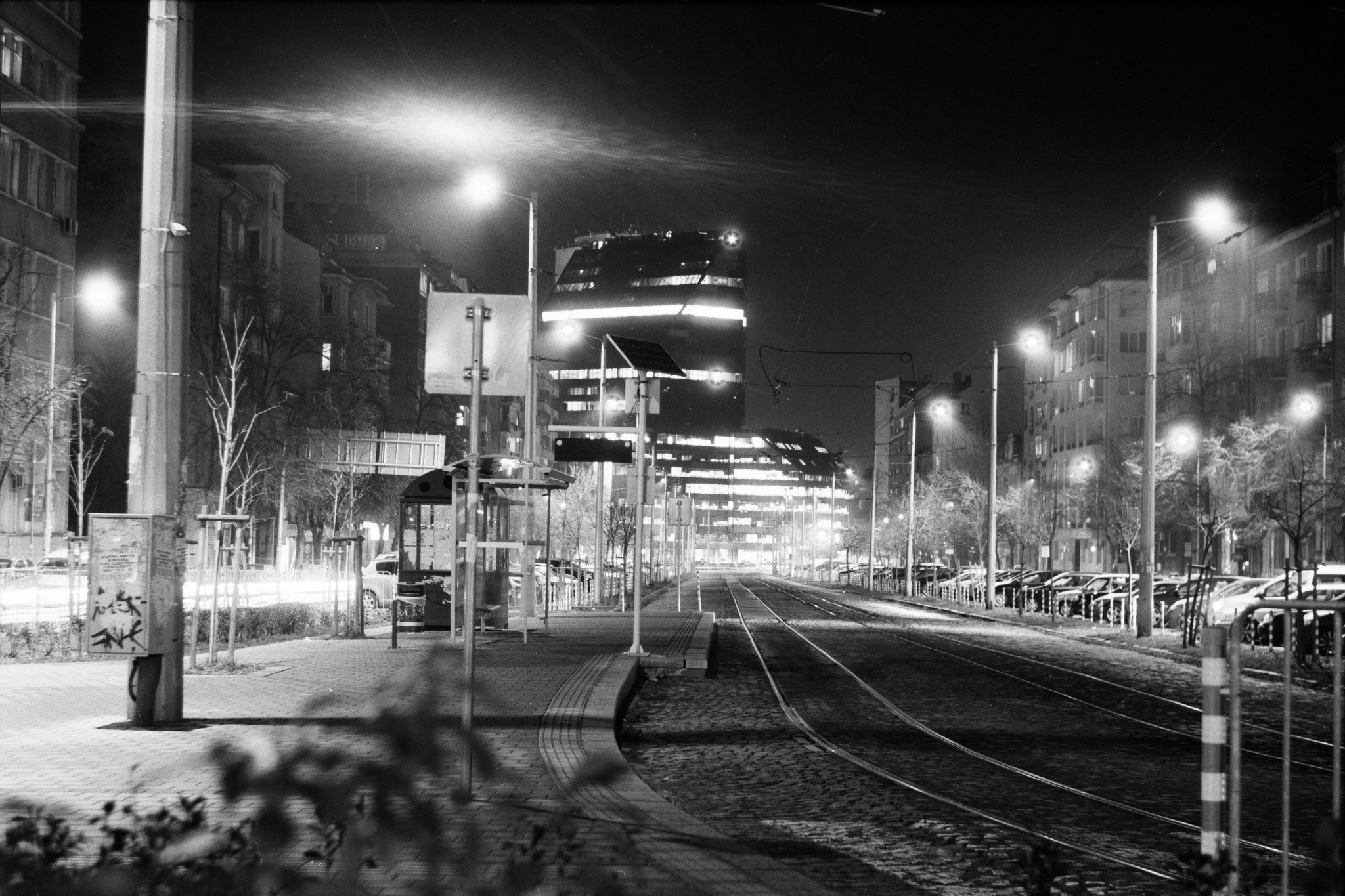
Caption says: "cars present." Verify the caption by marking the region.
[35,549,89,568]
[809,560,1345,659]
[362,549,705,610]
[0,556,35,574]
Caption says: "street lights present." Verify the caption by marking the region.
[1135,187,1245,641]
[460,180,539,641]
[828,467,853,584]
[986,322,1051,609]
[42,270,121,557]
[903,398,953,597]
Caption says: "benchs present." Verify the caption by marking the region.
[477,604,501,635]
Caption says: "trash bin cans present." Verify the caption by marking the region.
[396,581,425,633]
